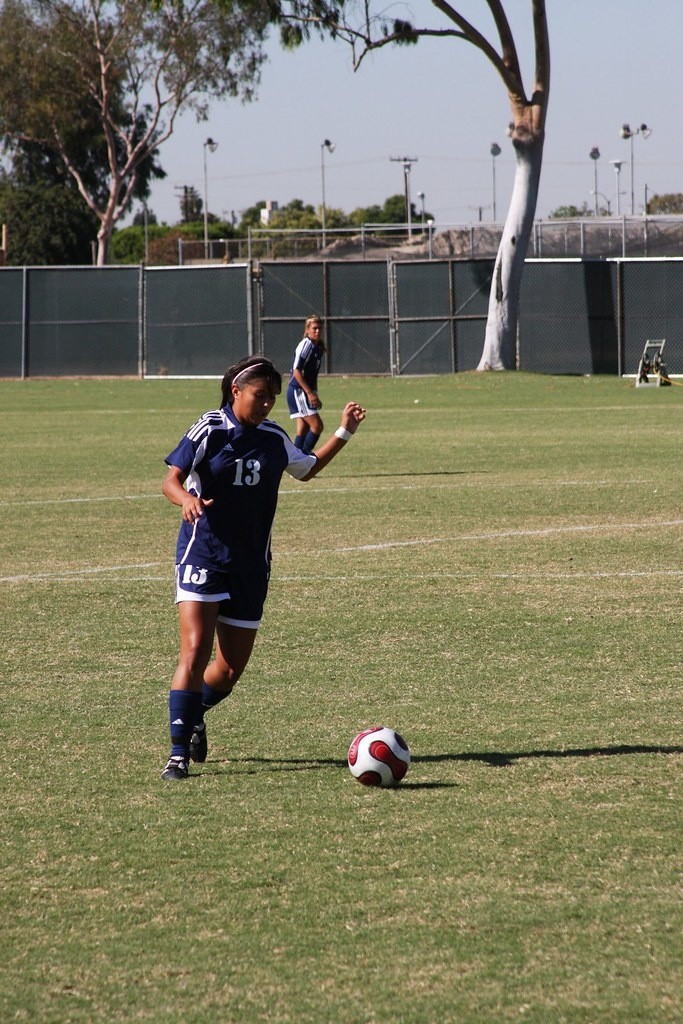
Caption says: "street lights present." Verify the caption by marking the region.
[491,143,501,222]
[611,157,624,214]
[321,139,335,247]
[203,137,219,265]
[619,122,653,214]
[427,220,434,260]
[589,147,601,218]
[591,191,630,258]
[416,191,424,236]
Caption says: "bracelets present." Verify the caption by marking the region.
[335,426,352,440]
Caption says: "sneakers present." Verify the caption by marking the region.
[161,756,189,780]
[189,723,207,763]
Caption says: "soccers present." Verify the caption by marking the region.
[346,724,411,789]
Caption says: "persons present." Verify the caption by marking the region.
[162,357,367,780]
[287,314,328,478]
